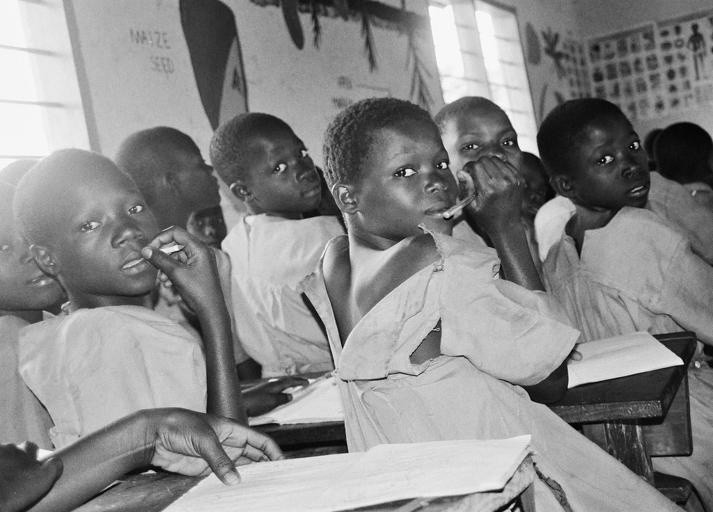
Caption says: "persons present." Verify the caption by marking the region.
[0,96,713,512]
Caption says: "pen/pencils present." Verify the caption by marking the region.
[160,244,186,254]
[443,192,478,219]
